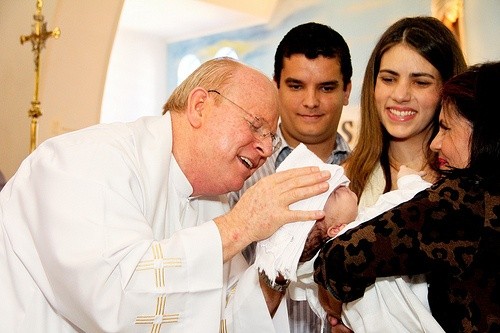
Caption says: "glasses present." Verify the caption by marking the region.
[208,90,282,154]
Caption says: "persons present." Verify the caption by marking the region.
[225,15,500,333]
[0,58,330,333]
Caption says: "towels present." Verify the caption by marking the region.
[253,143,350,288]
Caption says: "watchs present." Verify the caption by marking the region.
[258,267,290,291]
